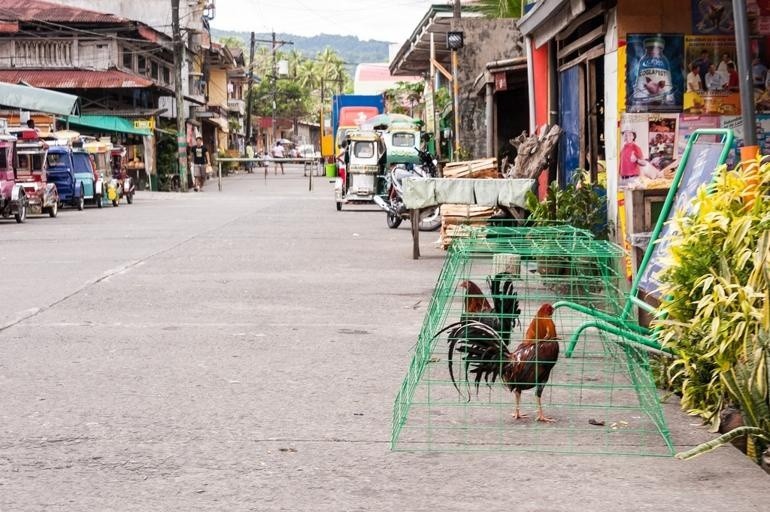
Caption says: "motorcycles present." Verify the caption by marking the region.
[373,145,443,232]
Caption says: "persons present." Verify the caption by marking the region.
[621,125,647,179]
[245,141,254,173]
[272,141,285,174]
[188,137,211,191]
[263,153,271,174]
[686,48,740,92]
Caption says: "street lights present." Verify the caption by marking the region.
[446,30,464,163]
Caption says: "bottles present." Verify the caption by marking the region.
[633,37,676,106]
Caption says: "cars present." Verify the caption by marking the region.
[329,112,425,212]
[0,126,136,224]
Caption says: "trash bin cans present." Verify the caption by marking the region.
[325,163,336,177]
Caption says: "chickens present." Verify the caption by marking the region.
[430,303,559,423]
[460,271,522,350]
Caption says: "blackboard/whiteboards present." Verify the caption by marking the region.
[636,143,724,302]
